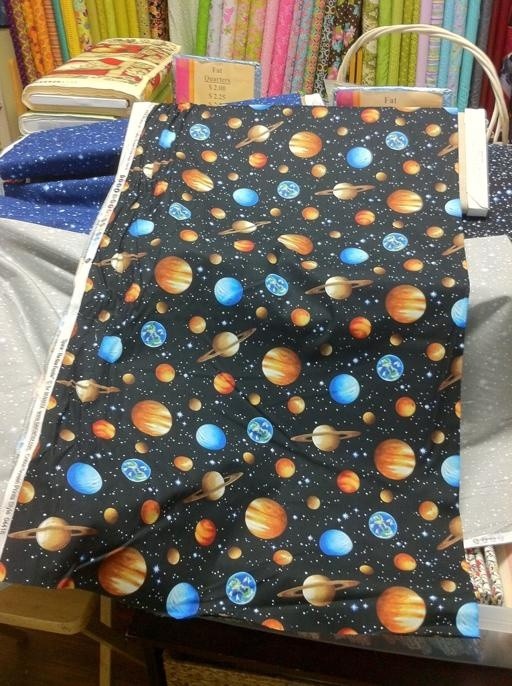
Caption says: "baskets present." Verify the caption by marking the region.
[336,22,510,144]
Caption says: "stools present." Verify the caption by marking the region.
[1,581,147,686]
